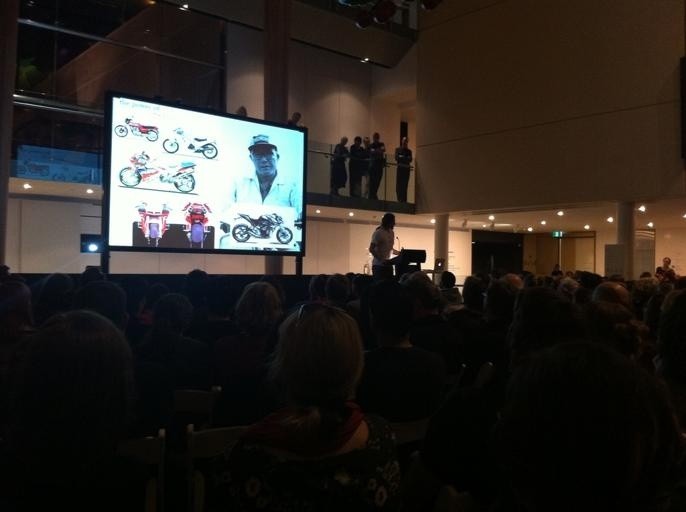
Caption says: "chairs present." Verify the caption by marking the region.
[423,258,445,285]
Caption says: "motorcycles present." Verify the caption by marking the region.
[175,202,214,251]
[113,117,159,142]
[132,202,170,248]
[117,150,197,193]
[161,127,219,160]
[231,212,294,246]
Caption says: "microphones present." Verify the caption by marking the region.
[396,237,400,250]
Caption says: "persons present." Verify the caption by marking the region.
[237,106,247,116]
[234,133,302,213]
[369,213,400,277]
[1,259,685,511]
[287,112,303,125]
[395,136,413,202]
[330,132,387,199]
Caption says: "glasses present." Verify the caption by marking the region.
[297,303,347,322]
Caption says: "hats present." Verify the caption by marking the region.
[248,133,278,151]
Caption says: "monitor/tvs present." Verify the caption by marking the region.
[103,88,308,258]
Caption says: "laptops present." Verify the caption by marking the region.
[425,258,445,271]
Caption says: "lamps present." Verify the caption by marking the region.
[87,243,98,252]
